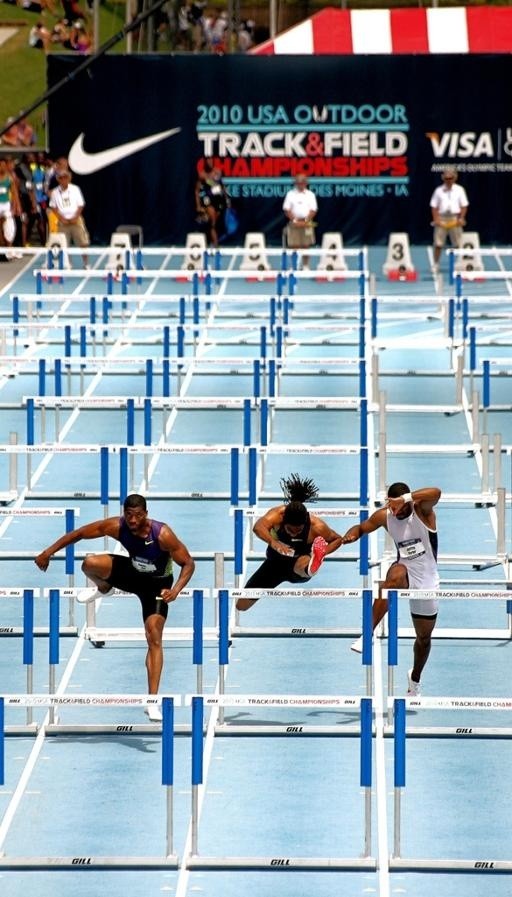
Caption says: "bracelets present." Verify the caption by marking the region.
[400,491,413,504]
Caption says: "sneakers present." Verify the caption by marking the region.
[142,692,165,723]
[405,667,422,697]
[75,583,116,604]
[350,631,374,653]
[307,535,328,577]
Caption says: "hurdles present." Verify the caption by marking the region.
[2,247,511,892]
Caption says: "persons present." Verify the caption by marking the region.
[341,481,441,696]
[3,1,94,54]
[197,169,230,248]
[280,171,319,278]
[234,472,343,610]
[33,492,197,722]
[45,154,90,271]
[155,0,258,54]
[0,112,56,247]
[428,167,470,265]
